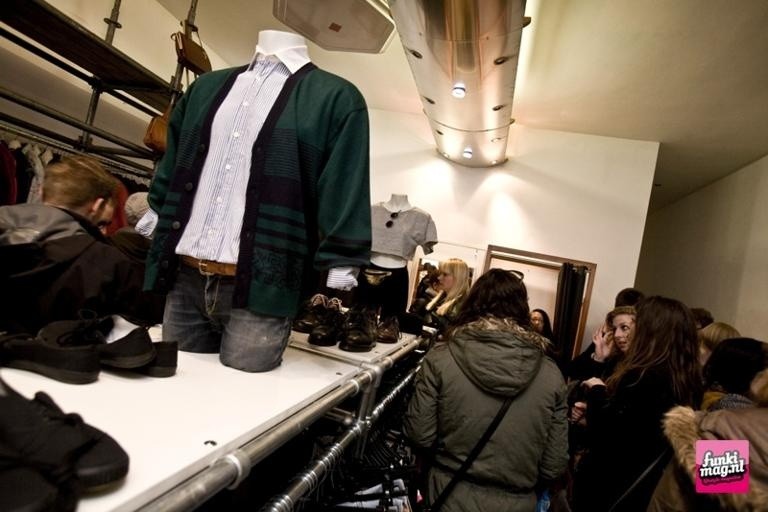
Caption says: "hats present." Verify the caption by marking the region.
[702,337,768,398]
[124,192,150,228]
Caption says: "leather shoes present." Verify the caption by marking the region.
[295,292,402,353]
[1,313,179,512]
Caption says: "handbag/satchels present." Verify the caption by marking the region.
[143,93,178,153]
[171,19,212,75]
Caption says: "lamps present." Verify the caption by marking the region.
[387,0,531,168]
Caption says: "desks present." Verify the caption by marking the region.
[0,307,436,512]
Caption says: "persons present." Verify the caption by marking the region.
[0,155,165,339]
[402,257,768,512]
[369,189,437,338]
[144,28,373,374]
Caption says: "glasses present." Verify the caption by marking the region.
[507,269,525,288]
[386,210,401,227]
[96,220,112,230]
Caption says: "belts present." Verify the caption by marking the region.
[174,252,238,278]
